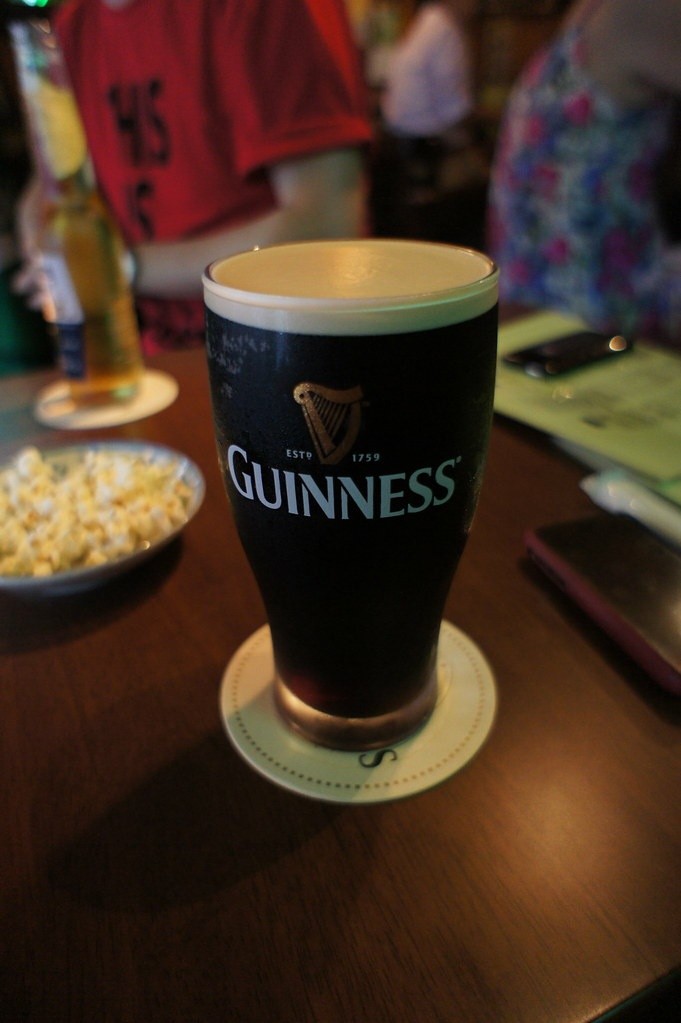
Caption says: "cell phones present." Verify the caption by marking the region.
[520,512,681,698]
[502,332,634,380]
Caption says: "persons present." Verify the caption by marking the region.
[362,1,477,190]
[13,1,372,358]
[487,0,681,344]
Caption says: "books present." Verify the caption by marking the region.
[494,308,680,506]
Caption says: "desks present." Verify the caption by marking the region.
[0,307,681,1023]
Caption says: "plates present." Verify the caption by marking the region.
[0,435,207,601]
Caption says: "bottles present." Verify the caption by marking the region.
[5,17,148,408]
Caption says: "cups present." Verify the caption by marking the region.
[200,232,506,753]
